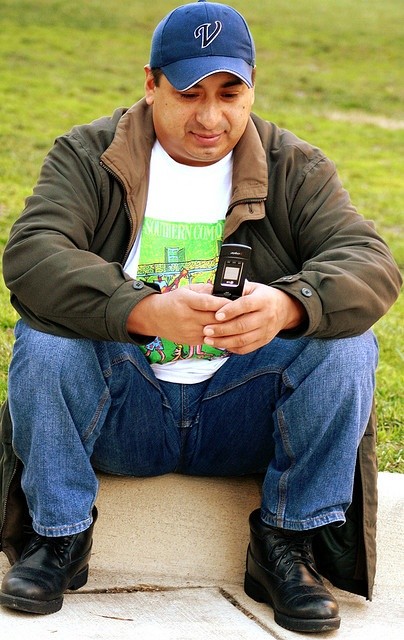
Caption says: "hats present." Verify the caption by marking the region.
[150,0,255,91]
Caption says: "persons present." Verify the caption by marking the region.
[0,0,403,634]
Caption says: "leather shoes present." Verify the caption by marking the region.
[244,508,341,632]
[0,505,98,616]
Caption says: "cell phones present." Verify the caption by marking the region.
[212,243,252,300]
